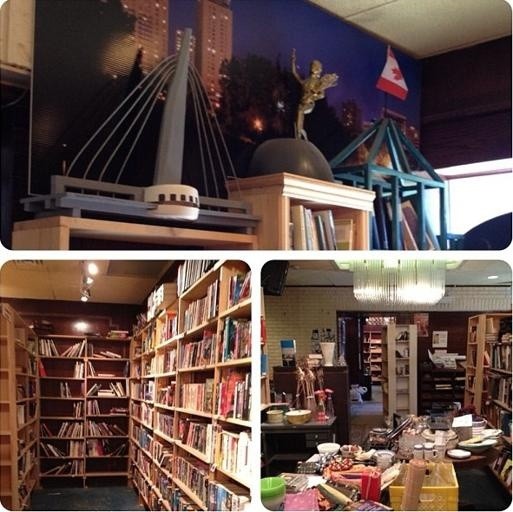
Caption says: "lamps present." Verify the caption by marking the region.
[79,261,99,302]
[333,260,468,306]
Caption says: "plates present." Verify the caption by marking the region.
[421,429,457,440]
[446,448,471,459]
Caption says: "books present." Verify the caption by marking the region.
[289,203,355,250]
[16,261,253,511]
[279,473,309,493]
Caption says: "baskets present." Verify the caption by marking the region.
[389,461,459,511]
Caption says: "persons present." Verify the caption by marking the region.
[291,48,326,141]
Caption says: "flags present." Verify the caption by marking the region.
[377,49,408,101]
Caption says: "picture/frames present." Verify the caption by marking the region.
[410,312,432,340]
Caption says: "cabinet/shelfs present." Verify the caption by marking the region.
[382,324,418,428]
[260,286,295,412]
[224,172,376,250]
[126,257,251,512]
[364,331,382,386]
[418,365,467,415]
[0,302,38,512]
[273,366,351,447]
[36,331,130,485]
[484,343,511,497]
[465,313,512,418]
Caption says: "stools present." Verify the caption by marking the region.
[268,455,311,477]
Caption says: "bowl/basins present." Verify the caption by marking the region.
[285,409,312,426]
[260,475,286,506]
[340,445,364,457]
[316,442,340,456]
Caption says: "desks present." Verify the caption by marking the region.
[261,414,337,477]
[382,410,504,508]
[11,215,258,249]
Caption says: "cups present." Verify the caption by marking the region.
[412,443,440,460]
